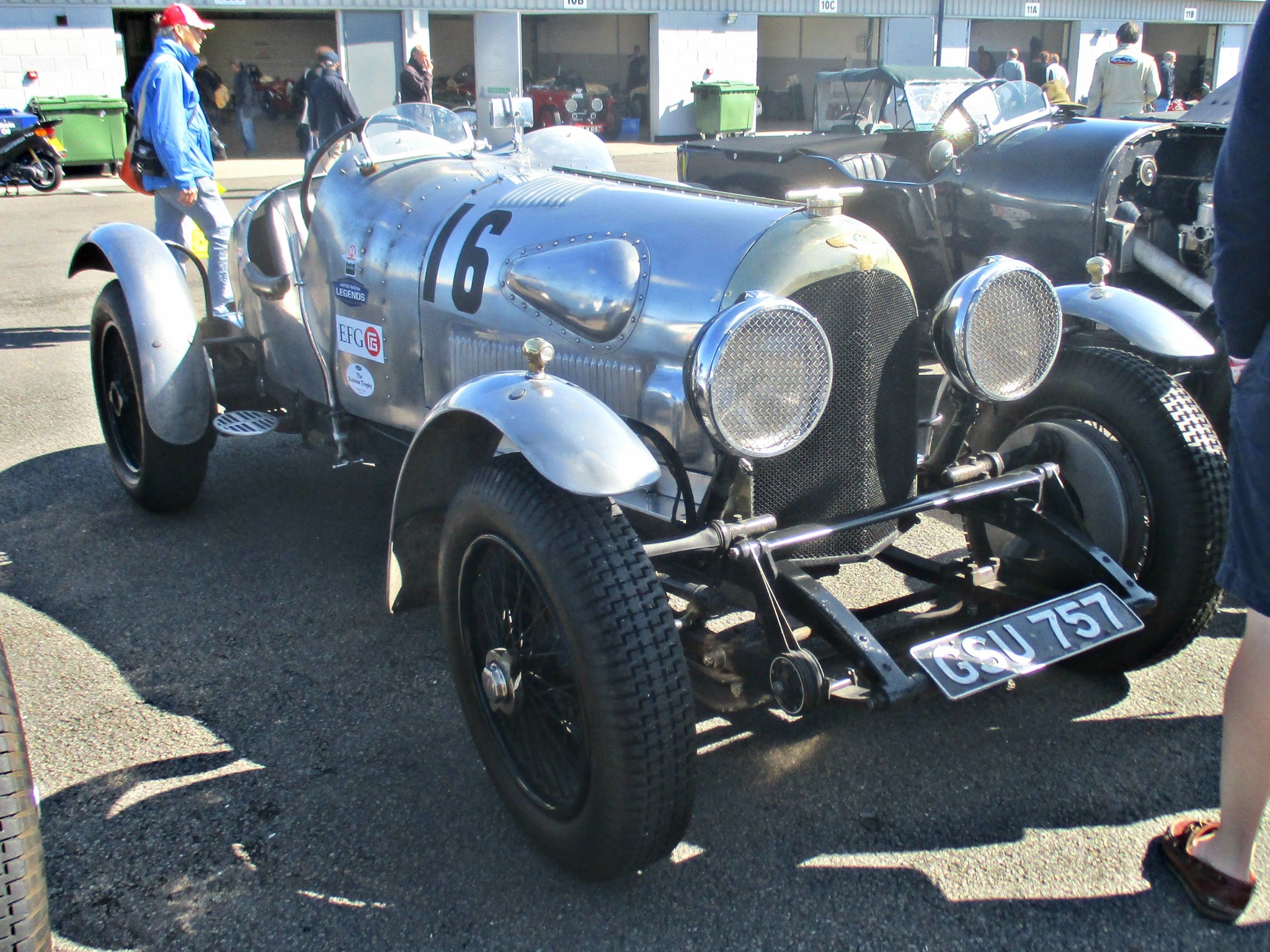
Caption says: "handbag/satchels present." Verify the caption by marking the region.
[120,148,155,196]
[129,139,166,178]
[214,83,229,108]
[304,133,323,175]
[248,102,262,118]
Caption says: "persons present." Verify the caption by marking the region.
[1153,0,1270,926]
[228,58,262,159]
[617,45,642,98]
[306,44,340,178]
[1082,22,1162,121]
[978,45,996,83]
[297,67,327,177]
[1152,50,1176,113]
[130,2,249,331]
[192,54,224,159]
[310,53,366,176]
[990,48,1027,122]
[1045,53,1071,117]
[1029,50,1051,113]
[398,44,435,138]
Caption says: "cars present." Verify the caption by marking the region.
[0,553,59,952]
[69,100,1239,884]
[247,67,300,121]
[432,64,624,144]
[675,77,1253,461]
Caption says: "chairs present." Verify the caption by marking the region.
[841,148,929,184]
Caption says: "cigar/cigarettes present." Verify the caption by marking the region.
[427,60,433,63]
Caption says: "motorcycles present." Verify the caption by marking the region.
[811,65,1006,133]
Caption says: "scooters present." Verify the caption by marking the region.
[0,119,69,197]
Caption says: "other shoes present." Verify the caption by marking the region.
[1159,818,1256,921]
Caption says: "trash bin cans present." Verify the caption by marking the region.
[28,95,128,176]
[690,80,759,140]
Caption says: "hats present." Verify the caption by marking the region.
[158,2,216,30]
[1040,80,1088,111]
[323,51,339,66]
